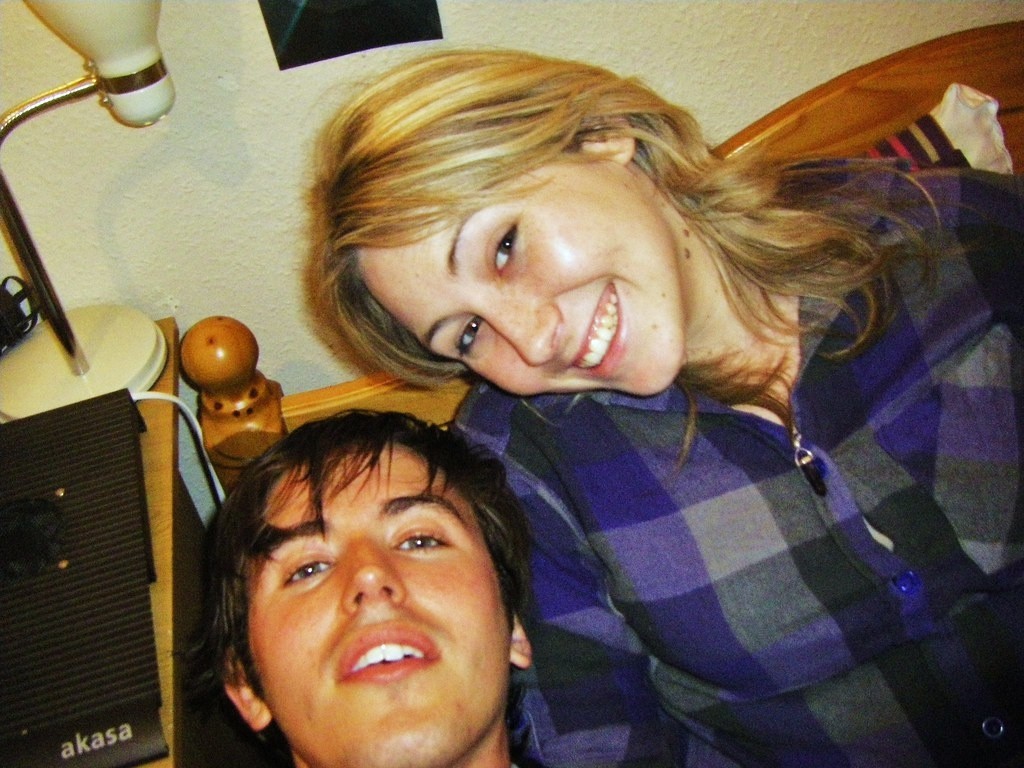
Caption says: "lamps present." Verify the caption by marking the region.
[0,0,177,425]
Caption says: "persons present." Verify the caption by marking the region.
[195,410,539,768]
[305,47,1024,768]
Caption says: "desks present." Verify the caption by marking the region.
[0,314,239,768]
